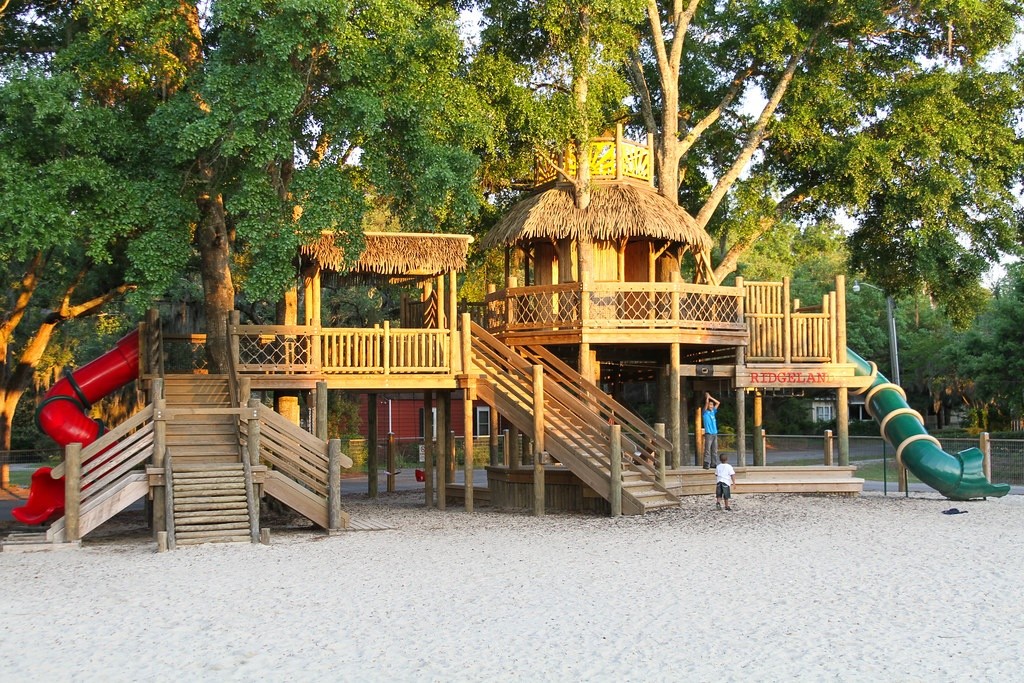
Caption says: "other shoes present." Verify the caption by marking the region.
[716,503,721,510]
[726,506,731,510]
[703,465,710,469]
[711,464,717,468]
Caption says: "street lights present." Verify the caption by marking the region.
[851,279,907,496]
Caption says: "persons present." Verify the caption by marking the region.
[702,392,720,469]
[714,454,736,511]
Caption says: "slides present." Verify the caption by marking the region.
[846,346,1012,502]
[9,324,142,527]
